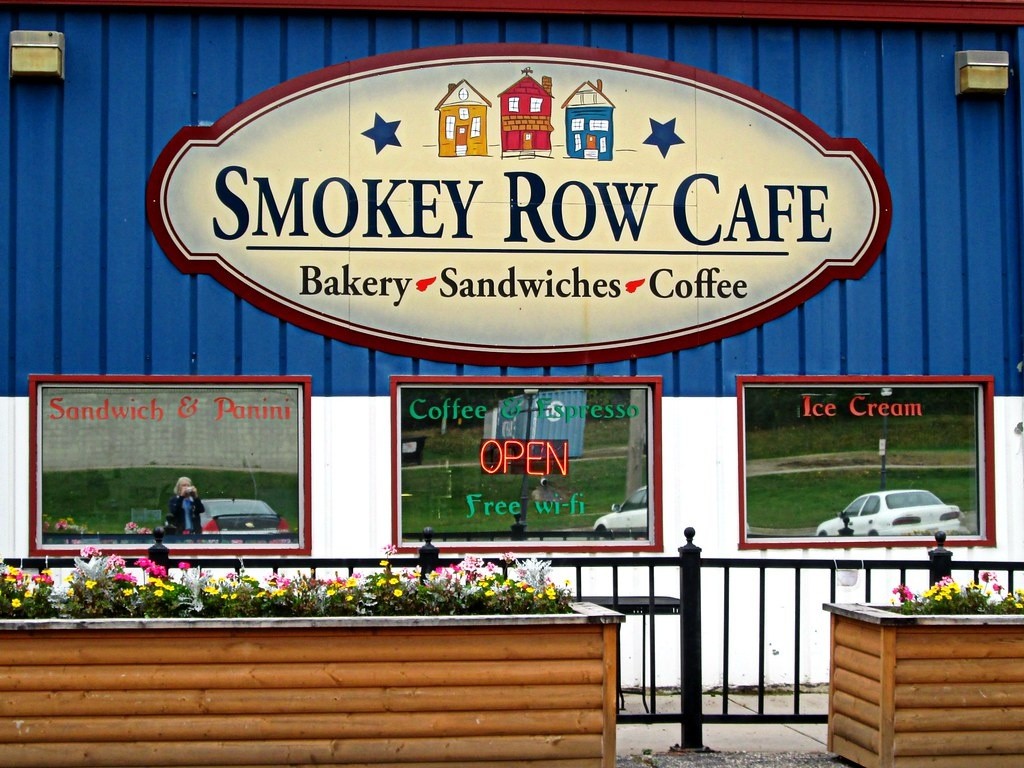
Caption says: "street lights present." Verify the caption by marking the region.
[510,390,550,531]
[879,389,894,490]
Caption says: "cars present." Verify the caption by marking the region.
[200,498,287,532]
[814,489,961,536]
[592,486,647,533]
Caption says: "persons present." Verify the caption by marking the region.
[169,477,205,534]
